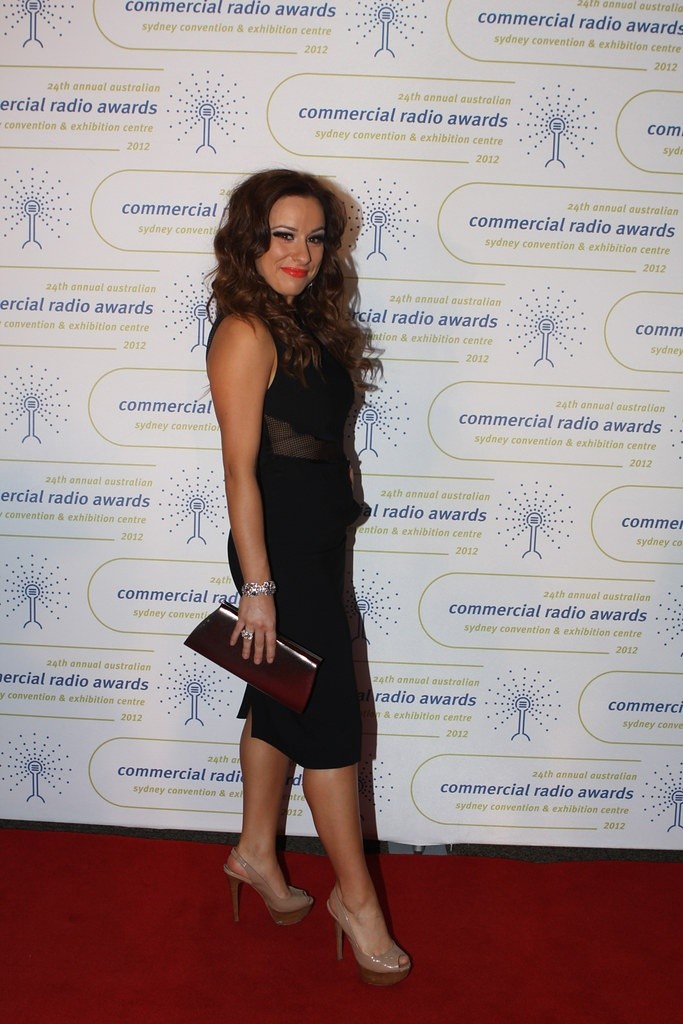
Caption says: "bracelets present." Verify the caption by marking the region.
[242,581,277,597]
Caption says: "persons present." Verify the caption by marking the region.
[207,172,416,985]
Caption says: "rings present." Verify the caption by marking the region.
[241,629,254,640]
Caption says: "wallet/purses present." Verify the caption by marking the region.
[182,602,322,714]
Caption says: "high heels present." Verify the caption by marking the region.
[221,847,412,985]
